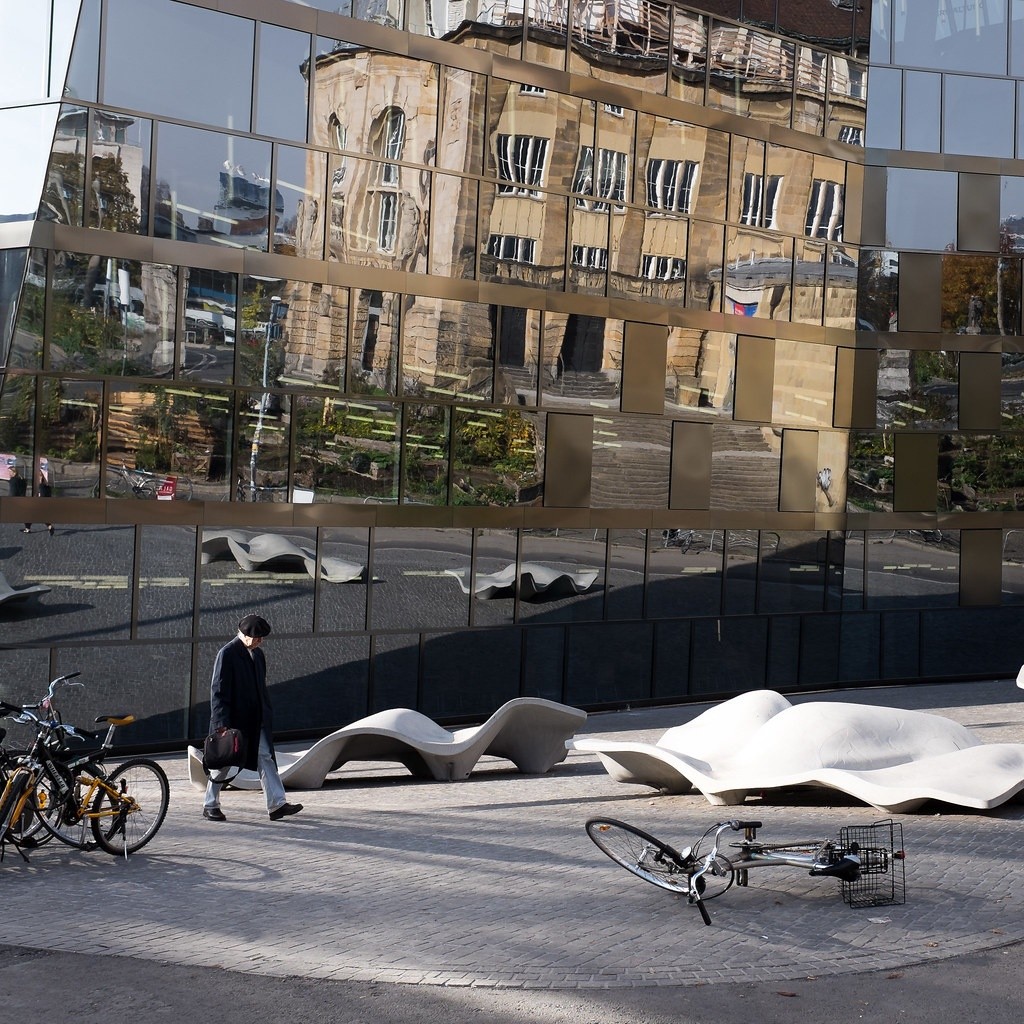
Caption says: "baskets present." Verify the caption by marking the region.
[839,818,905,909]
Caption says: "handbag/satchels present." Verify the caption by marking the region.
[202,725,245,784]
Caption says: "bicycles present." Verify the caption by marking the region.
[584,815,906,926]
[0,670,170,863]
[911,528,945,542]
[664,529,700,552]
[220,475,247,502]
[105,459,194,503]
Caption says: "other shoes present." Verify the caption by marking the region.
[19,529,30,533]
[49,526,54,536]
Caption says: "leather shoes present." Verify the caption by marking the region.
[203,807,226,821]
[269,803,303,820]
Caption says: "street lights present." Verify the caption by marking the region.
[117,268,130,376]
[263,296,282,388]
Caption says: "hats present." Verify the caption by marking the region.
[238,614,271,638]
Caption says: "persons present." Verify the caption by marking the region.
[203,615,303,821]
